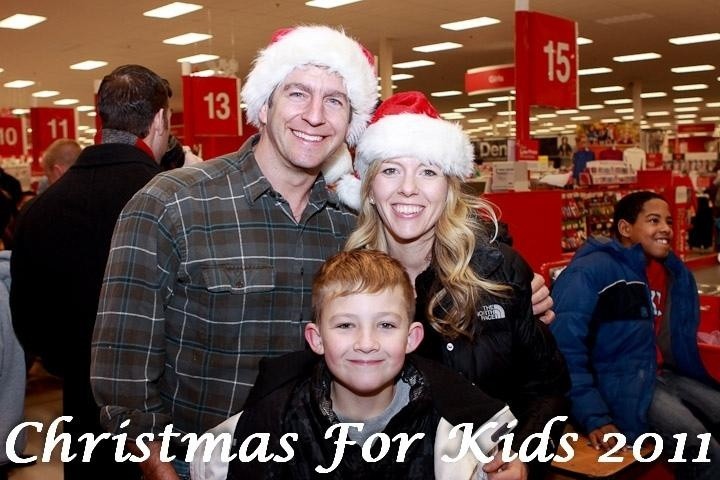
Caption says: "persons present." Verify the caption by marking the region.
[91,23,555,480]
[544,191,720,479]
[558,136,571,151]
[0,136,208,480]
[10,63,170,480]
[226,249,502,480]
[572,136,597,186]
[597,137,623,161]
[343,90,571,480]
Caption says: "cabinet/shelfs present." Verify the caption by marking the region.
[471,125,720,273]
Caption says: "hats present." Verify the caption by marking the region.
[239,24,379,147]
[322,91,475,211]
